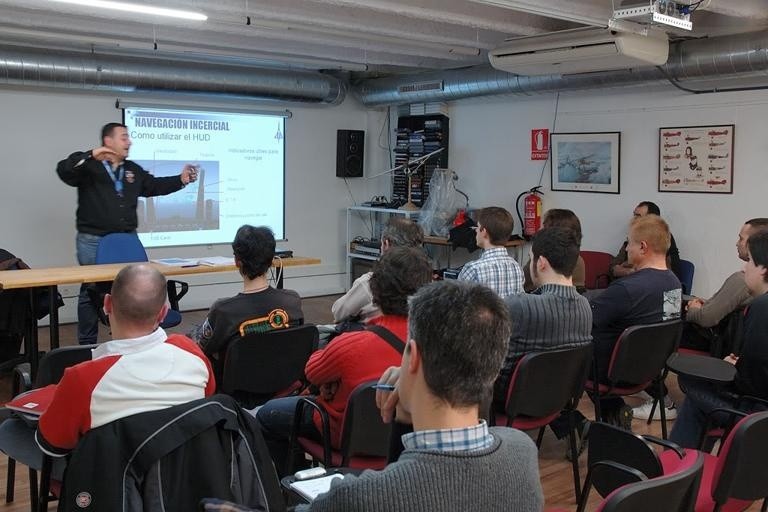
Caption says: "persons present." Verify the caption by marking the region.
[295,280,546,512]
[608,201,681,279]
[1,264,217,484]
[254,246,436,472]
[55,122,198,344]
[186,223,305,390]
[663,231,768,455]
[546,213,682,463]
[661,218,767,407]
[484,227,595,417]
[457,207,526,297]
[523,208,587,291]
[314,216,425,324]
[0,249,35,406]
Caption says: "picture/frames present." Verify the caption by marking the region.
[550,131,621,195]
[659,124,736,196]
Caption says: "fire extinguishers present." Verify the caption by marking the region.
[516,184,544,241]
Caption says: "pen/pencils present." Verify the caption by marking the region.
[372,385,395,391]
[181,265,199,268]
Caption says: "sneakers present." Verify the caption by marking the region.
[631,397,680,422]
[563,414,594,462]
[603,405,632,434]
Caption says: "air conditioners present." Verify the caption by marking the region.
[488,24,669,76]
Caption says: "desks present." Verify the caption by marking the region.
[418,234,522,253]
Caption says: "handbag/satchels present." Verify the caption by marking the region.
[326,319,368,347]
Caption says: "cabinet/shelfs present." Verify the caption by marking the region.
[344,201,452,290]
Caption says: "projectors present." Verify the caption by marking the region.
[614,0,691,26]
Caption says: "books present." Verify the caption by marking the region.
[392,118,441,202]
[290,473,345,502]
[149,256,235,269]
[4,384,58,416]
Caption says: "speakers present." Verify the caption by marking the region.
[337,129,365,177]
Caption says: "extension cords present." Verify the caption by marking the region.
[365,200,387,204]
